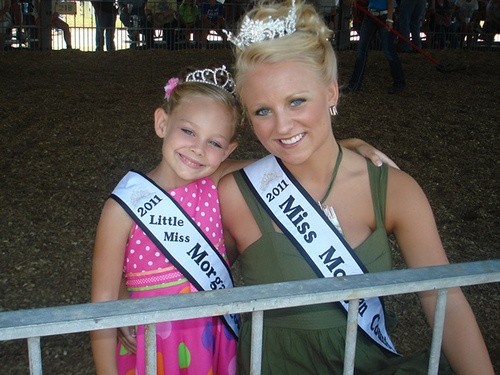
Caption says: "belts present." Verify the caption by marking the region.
[371,7,395,16]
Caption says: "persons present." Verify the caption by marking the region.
[88,63,401,374]
[217,0,496,375]
[340,1,406,95]
[1,1,500,52]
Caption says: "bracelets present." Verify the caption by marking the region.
[386,19,393,23]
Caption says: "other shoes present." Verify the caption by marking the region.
[342,87,357,94]
[385,86,404,93]
